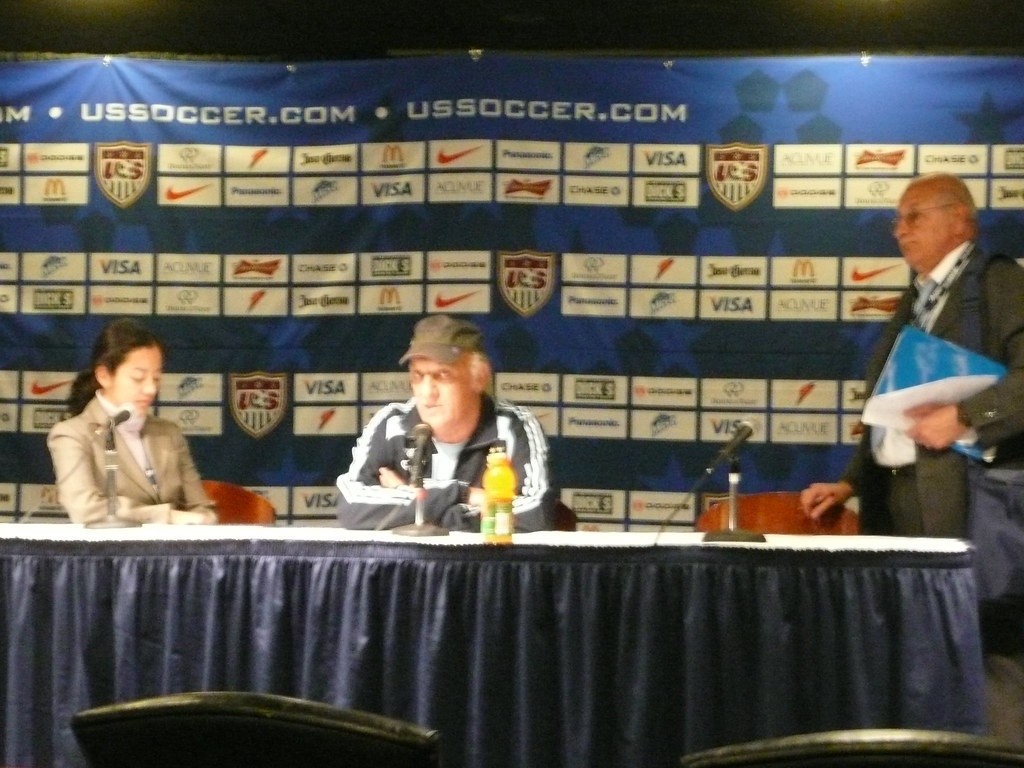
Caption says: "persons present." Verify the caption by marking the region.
[338,315,560,532]
[47,318,219,529]
[799,172,1024,535]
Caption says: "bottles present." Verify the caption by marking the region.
[481,453,515,548]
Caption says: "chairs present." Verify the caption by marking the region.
[552,499,576,531]
[201,480,274,527]
[69,691,442,768]
[695,493,859,536]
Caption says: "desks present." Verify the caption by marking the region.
[0,522,982,768]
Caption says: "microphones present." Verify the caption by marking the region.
[705,421,756,474]
[407,424,434,489]
[94,402,138,436]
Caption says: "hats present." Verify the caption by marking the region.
[398,315,483,366]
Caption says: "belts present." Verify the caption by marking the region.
[876,467,916,481]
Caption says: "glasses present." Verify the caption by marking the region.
[888,203,953,237]
